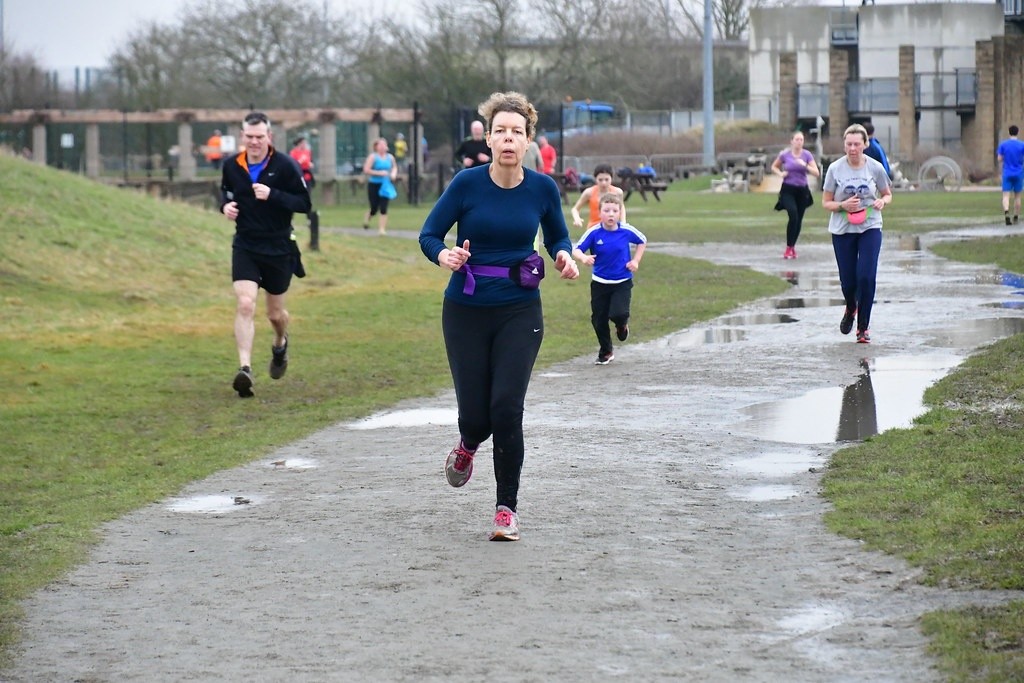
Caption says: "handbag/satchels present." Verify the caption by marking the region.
[462,250,545,294]
[840,206,872,225]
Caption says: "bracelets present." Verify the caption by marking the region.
[839,203,842,209]
[807,164,808,166]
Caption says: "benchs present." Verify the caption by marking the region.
[565,186,592,192]
[612,183,667,190]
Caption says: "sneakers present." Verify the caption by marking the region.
[784,244,796,260]
[445,437,481,487]
[232,372,255,398]
[840,302,859,334]
[617,321,629,342]
[489,504,519,542]
[595,348,613,364]
[856,328,871,343]
[269,330,288,379]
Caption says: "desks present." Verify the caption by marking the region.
[546,173,584,206]
[618,173,661,203]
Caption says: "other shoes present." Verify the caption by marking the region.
[1013,216,1019,223]
[1005,213,1012,226]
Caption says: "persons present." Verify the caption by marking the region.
[863,122,890,174]
[823,124,894,343]
[836,358,877,441]
[779,271,806,324]
[538,135,556,176]
[455,120,492,169]
[524,142,544,171]
[288,136,318,251]
[572,163,626,230]
[422,137,428,161]
[206,129,223,168]
[573,196,647,365]
[418,91,579,541]
[364,138,398,235]
[998,126,1024,225]
[219,112,312,398]
[394,133,408,162]
[771,130,820,259]
